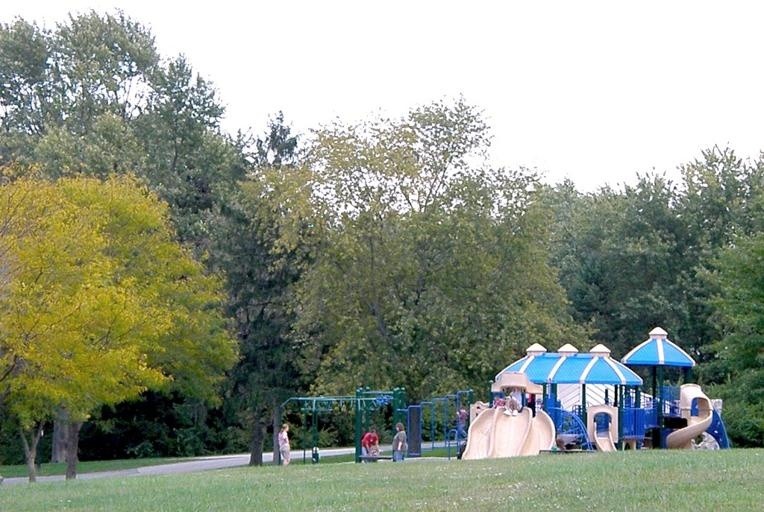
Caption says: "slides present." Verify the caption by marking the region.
[594,435,617,451]
[666,412,713,449]
[461,406,556,460]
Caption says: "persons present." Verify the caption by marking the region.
[456,403,470,427]
[361,429,380,464]
[367,443,380,463]
[391,422,408,461]
[554,415,581,453]
[277,423,291,465]
[490,384,523,416]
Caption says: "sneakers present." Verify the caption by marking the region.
[503,410,510,415]
[512,410,518,415]
